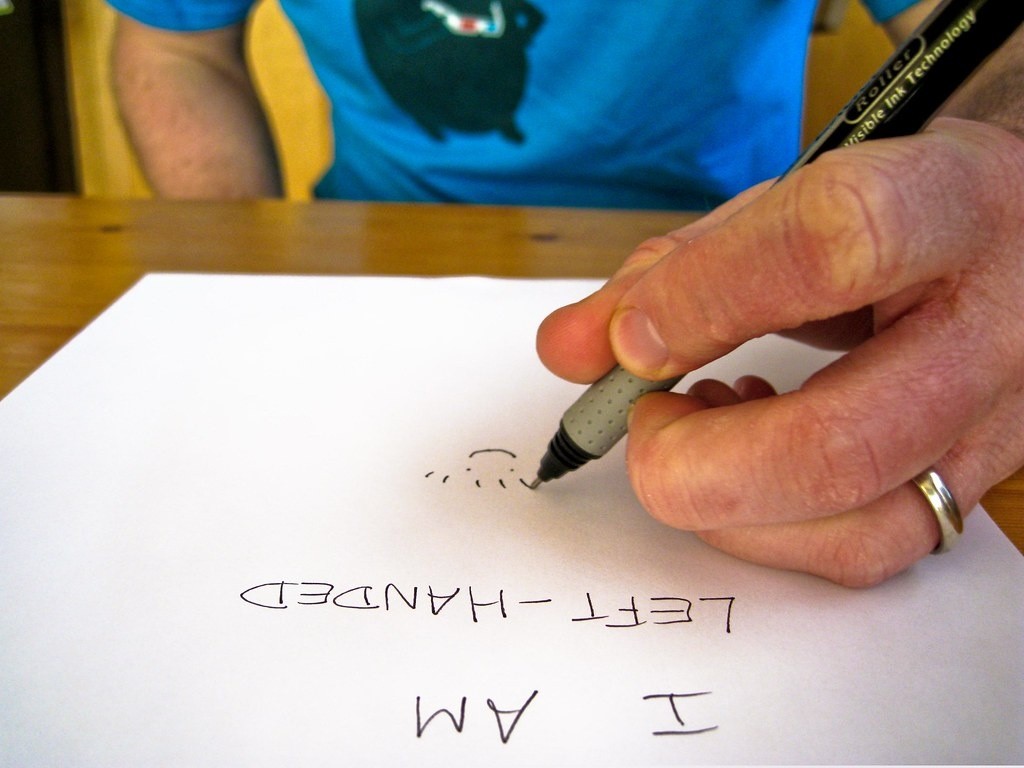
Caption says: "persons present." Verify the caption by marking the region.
[100,0,1023,590]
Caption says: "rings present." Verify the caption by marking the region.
[906,468,964,554]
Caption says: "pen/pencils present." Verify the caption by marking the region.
[529,0,1024,489]
